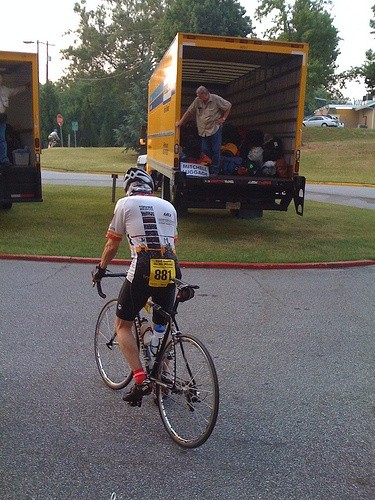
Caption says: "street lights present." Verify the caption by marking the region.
[22,39,40,61]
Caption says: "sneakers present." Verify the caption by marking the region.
[123,378,151,402]
[168,347,176,360]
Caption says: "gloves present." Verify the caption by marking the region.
[92,264,108,281]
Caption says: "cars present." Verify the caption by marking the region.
[302,115,338,127]
[324,115,345,128]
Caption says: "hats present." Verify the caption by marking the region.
[53,129,58,132]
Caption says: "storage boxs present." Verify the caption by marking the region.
[13,150,30,165]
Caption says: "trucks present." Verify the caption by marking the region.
[0,50,44,208]
[137,33,308,220]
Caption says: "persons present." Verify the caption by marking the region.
[48,128,60,148]
[176,85,232,174]
[91,166,182,403]
[0,70,31,167]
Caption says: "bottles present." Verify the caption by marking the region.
[140,317,154,346]
[150,324,165,362]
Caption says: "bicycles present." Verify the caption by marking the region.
[47,140,61,149]
[88,270,221,449]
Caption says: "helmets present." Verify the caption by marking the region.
[124,168,154,196]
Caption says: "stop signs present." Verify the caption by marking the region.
[56,114,64,126]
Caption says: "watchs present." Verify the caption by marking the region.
[222,118,225,121]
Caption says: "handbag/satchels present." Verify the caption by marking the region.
[220,136,285,178]
